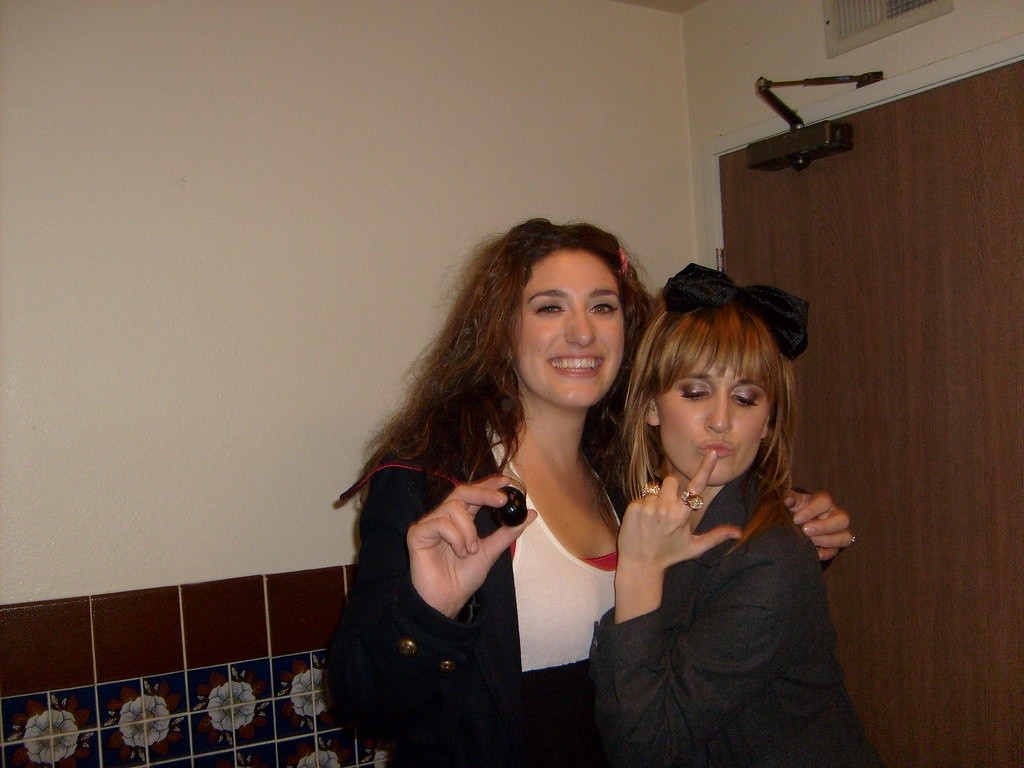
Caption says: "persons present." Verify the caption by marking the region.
[324,218,854,768]
[588,263,886,768]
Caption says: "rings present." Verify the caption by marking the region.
[642,481,660,499]
[852,536,856,543]
[681,490,704,510]
